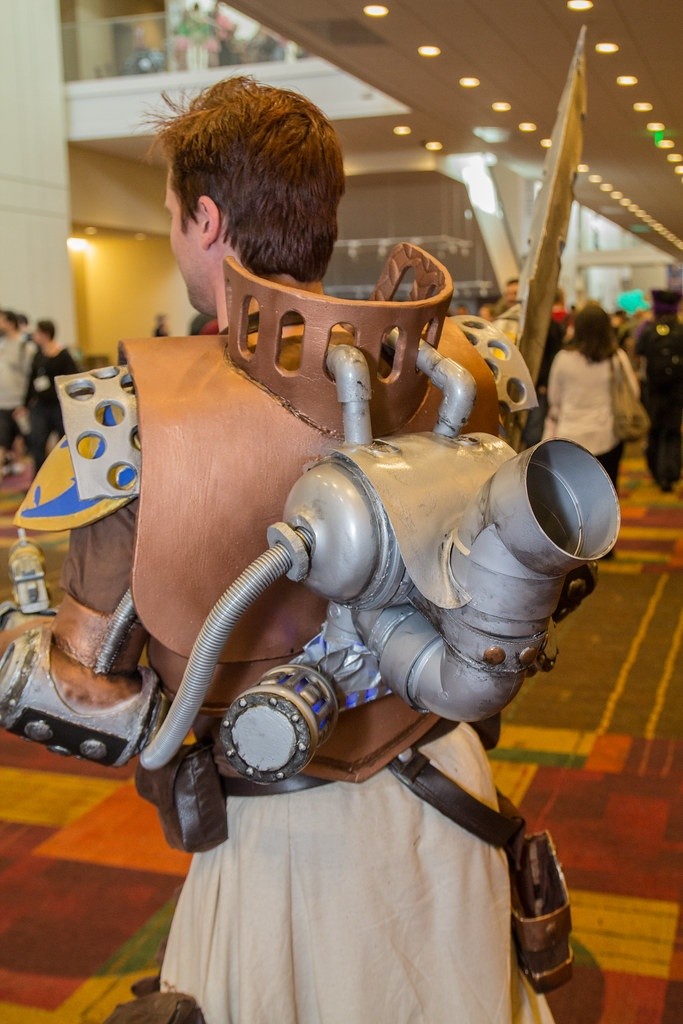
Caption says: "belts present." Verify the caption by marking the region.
[217,716,461,797]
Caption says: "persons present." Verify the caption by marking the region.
[0,76,557,1023]
[458,277,519,320]
[153,315,167,337]
[0,311,80,497]
[521,286,683,558]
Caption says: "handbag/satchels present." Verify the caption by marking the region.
[610,354,650,443]
